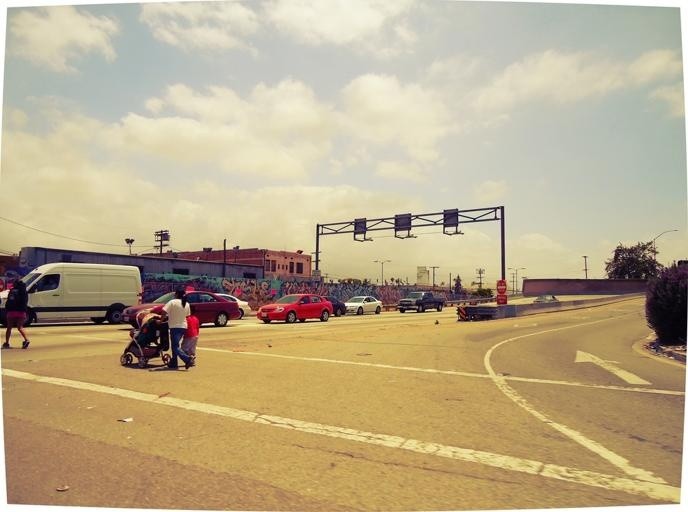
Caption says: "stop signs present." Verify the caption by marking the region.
[496,280,506,296]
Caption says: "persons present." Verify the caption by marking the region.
[180,308,199,368]
[1,277,30,349]
[41,276,58,290]
[155,288,193,368]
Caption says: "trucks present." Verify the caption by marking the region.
[0,259,145,329]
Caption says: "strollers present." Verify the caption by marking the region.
[119,312,174,371]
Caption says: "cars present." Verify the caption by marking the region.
[321,295,347,318]
[202,292,251,319]
[257,293,335,324]
[121,290,240,330]
[344,295,384,316]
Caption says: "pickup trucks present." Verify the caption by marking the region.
[395,291,448,313]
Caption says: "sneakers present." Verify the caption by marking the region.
[23,340,30,348]
[186,357,193,368]
[169,364,177,370]
[2,342,9,349]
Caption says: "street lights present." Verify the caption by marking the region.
[508,265,527,296]
[124,237,134,256]
[372,259,392,288]
[652,228,679,275]
[429,266,439,287]
[582,255,590,279]
[232,245,241,263]
